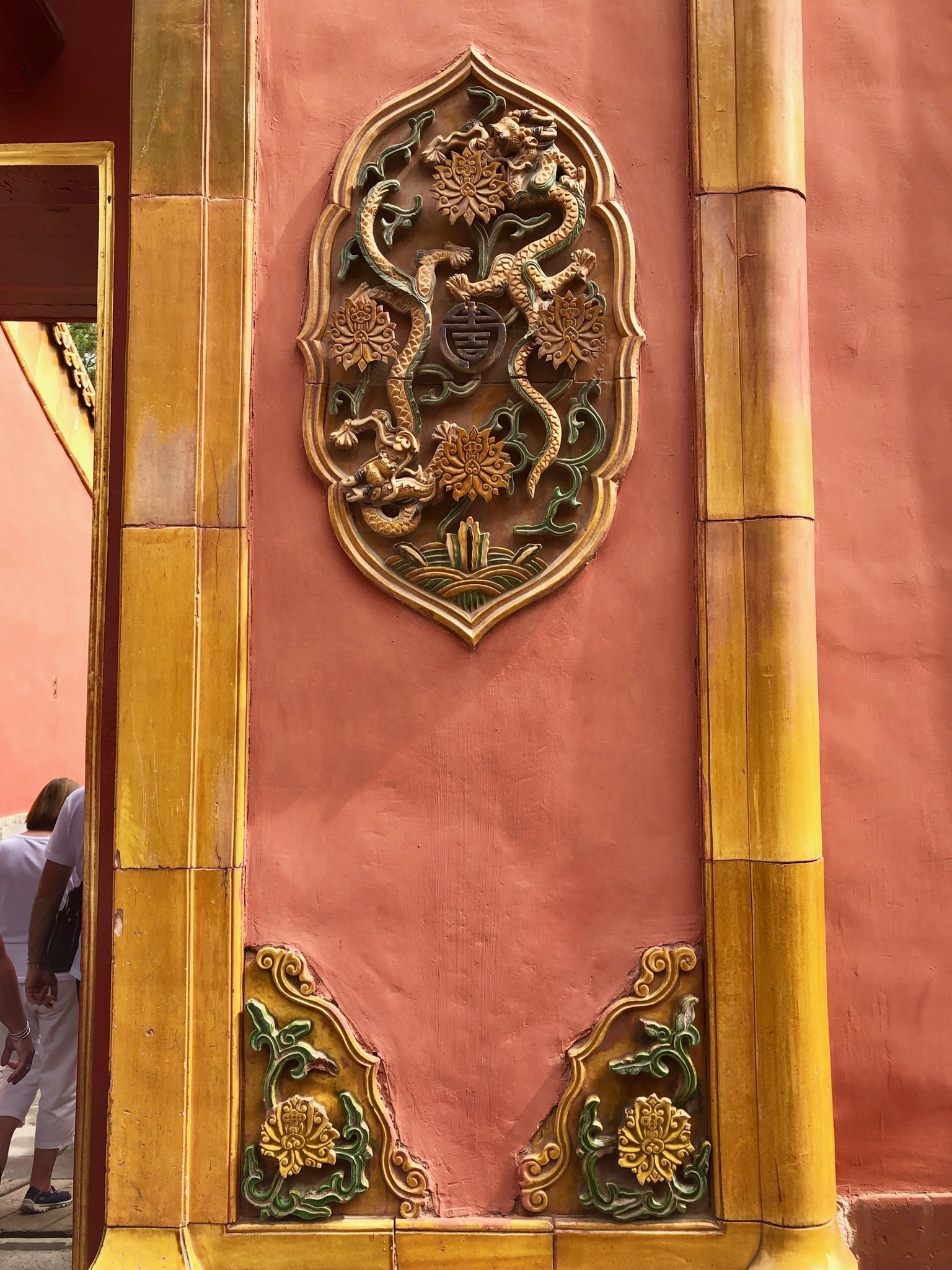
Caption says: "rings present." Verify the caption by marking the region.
[25,1065,31,1070]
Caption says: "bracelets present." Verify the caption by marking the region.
[27,960,45,970]
[9,1020,30,1041]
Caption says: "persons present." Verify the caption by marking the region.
[24,784,84,1010]
[0,777,83,1213]
[0,934,35,1086]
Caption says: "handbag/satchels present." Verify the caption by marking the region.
[43,887,83,973]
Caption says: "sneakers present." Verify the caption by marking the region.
[18,1185,73,1214]
